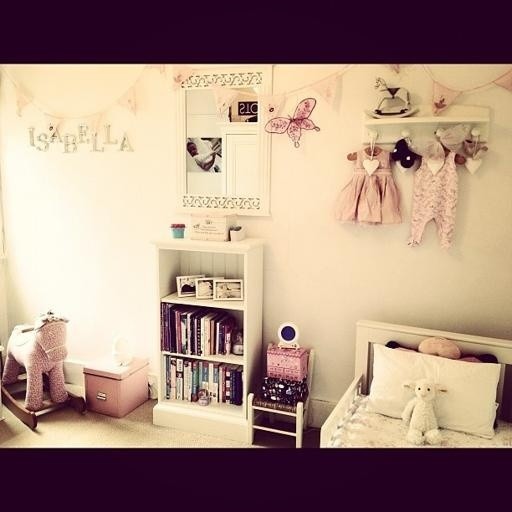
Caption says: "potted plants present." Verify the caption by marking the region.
[230,225,244,242]
[169,224,186,238]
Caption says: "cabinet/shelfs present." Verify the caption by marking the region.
[153,239,263,444]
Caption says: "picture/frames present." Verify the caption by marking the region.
[173,64,273,217]
[176,274,243,300]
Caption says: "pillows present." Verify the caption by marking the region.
[386,341,505,430]
[366,343,502,439]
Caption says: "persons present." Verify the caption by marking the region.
[187,142,221,172]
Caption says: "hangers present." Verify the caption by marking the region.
[347,132,396,162]
[414,135,467,165]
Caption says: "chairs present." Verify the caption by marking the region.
[248,344,316,449]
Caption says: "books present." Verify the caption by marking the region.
[160,302,243,406]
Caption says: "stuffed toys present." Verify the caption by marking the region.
[402,379,449,445]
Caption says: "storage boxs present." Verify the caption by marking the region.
[267,347,308,382]
[83,359,150,418]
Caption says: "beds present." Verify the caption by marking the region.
[320,319,512,448]
